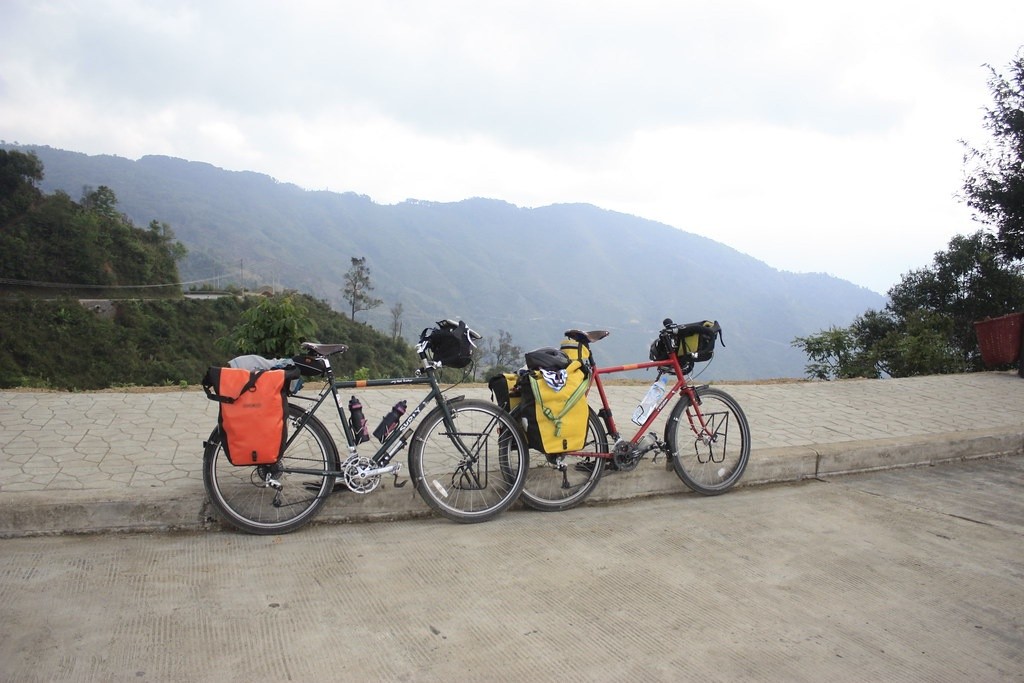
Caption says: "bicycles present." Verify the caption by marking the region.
[489,320,751,513]
[200,317,530,537]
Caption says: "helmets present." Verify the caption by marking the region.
[525,346,573,372]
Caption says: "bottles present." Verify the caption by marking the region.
[631,374,668,424]
[348,395,370,442]
[637,432,658,451]
[372,400,407,442]
